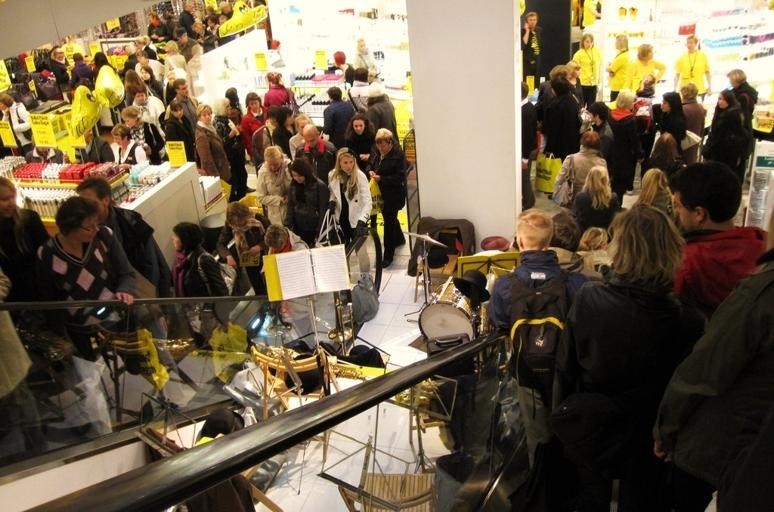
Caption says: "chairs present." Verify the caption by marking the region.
[91,324,201,427]
[405,216,475,306]
[338,471,438,512]
[245,344,343,476]
[173,411,289,512]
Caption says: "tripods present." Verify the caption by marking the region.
[413,407,437,475]
[262,298,370,494]
[404,254,431,316]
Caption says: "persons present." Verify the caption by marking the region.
[484,162,773,510]
[2,0,407,457]
[522,1,760,186]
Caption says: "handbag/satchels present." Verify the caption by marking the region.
[351,274,380,324]
[681,130,701,150]
[315,209,344,248]
[552,178,574,206]
[133,270,157,319]
[535,152,562,192]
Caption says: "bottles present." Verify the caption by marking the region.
[0,156,168,219]
[311,97,330,112]
[295,72,314,80]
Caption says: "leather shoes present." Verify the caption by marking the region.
[395,238,405,246]
[381,257,393,268]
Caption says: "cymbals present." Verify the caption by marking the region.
[402,230,446,249]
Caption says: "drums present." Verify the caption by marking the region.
[478,301,496,337]
[418,281,477,342]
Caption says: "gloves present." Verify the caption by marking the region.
[356,221,366,236]
[327,201,335,214]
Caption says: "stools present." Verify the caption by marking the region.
[480,236,512,252]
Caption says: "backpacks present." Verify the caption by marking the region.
[505,269,577,390]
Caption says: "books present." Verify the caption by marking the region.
[456,249,519,279]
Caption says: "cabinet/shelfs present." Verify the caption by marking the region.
[255,66,349,133]
[0,158,231,275]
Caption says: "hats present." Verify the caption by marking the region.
[453,270,491,303]
[367,82,385,98]
[428,246,449,268]
